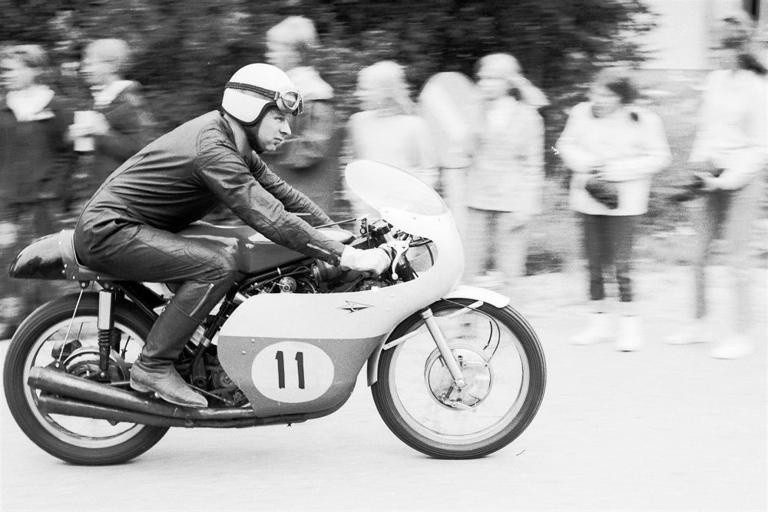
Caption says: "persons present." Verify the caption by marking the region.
[1,38,159,325]
[259,16,338,222]
[554,67,673,318]
[688,16,767,321]
[73,62,391,411]
[341,52,552,278]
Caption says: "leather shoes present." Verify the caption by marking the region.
[129,355,209,409]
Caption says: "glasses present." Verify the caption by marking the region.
[225,81,303,115]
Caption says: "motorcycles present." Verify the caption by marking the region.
[3,160,549,466]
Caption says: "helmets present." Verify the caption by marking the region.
[220,61,304,126]
